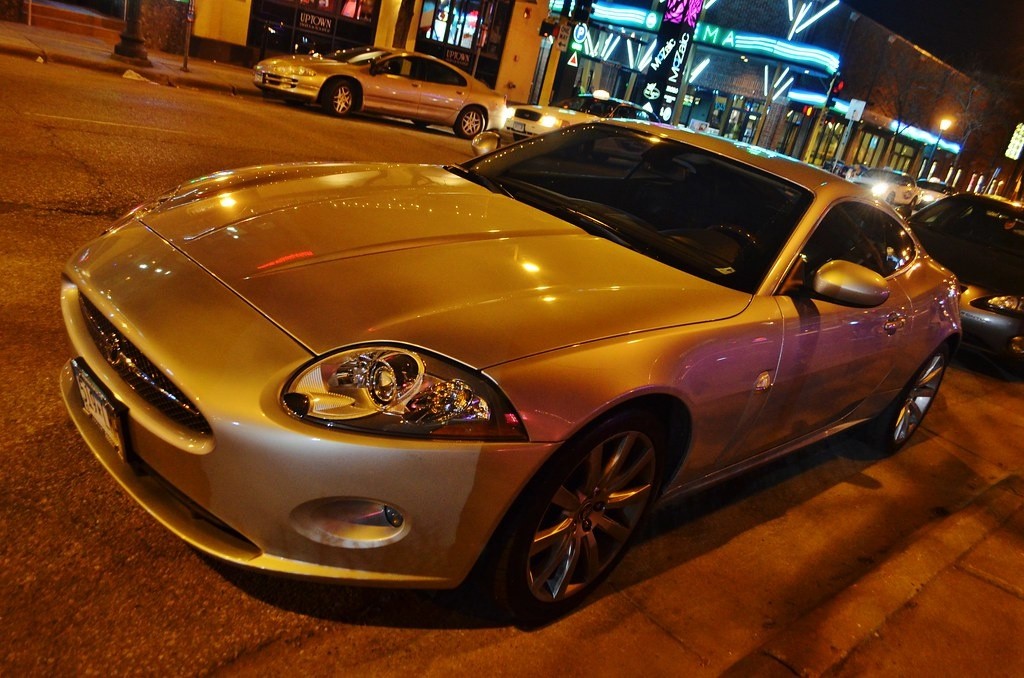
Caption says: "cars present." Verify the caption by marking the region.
[904,191,1024,381]
[58,120,963,628]
[916,176,952,207]
[503,90,662,141]
[253,46,508,141]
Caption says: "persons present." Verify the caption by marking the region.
[573,79,583,96]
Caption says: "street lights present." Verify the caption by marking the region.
[921,117,954,179]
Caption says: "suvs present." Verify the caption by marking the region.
[850,166,918,216]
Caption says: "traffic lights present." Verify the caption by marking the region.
[825,71,843,110]
